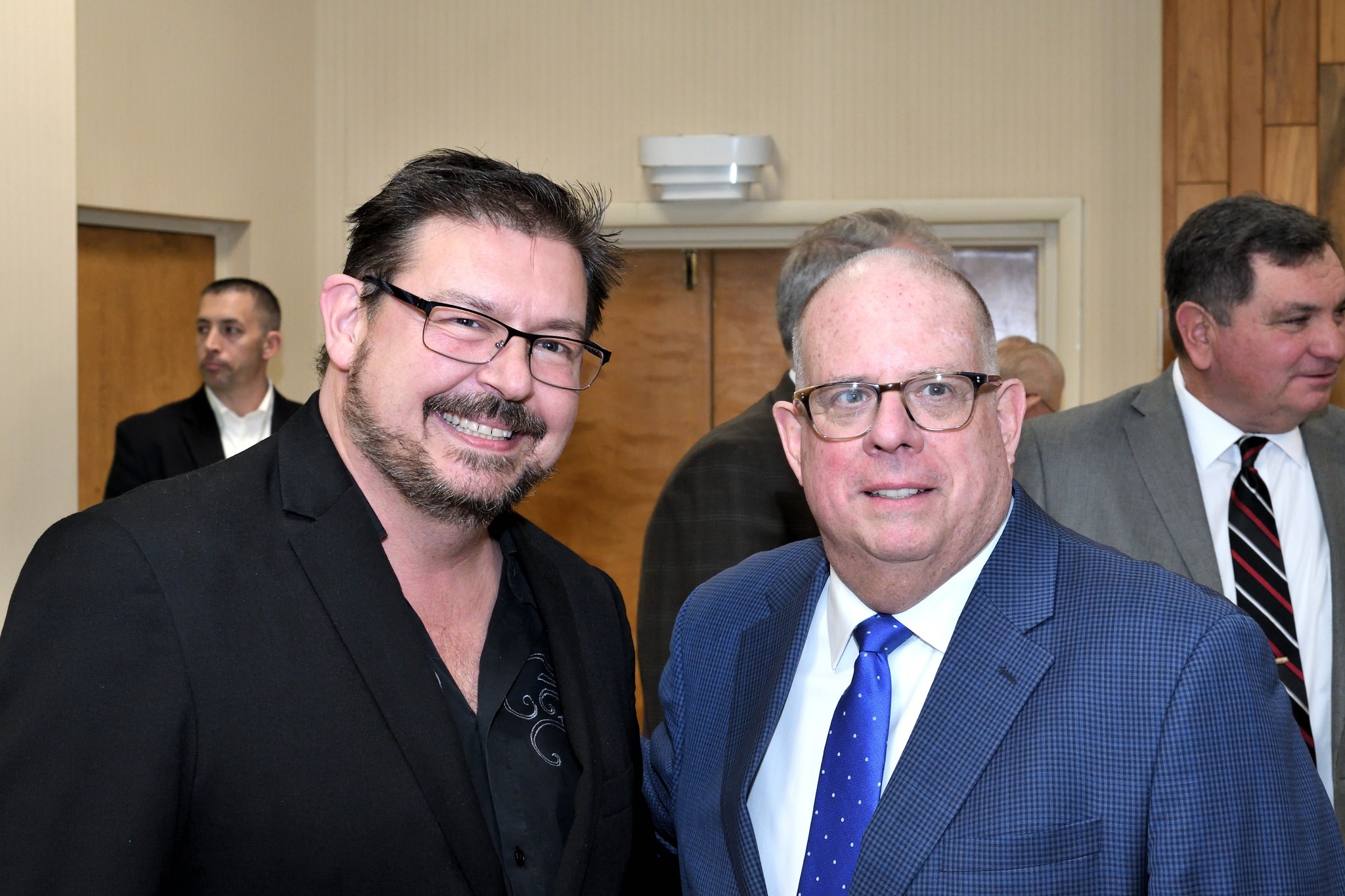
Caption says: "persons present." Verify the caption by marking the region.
[0,147,681,896]
[997,336,1065,419]
[1013,190,1345,838]
[643,247,1345,896]
[634,207,965,743]
[103,277,304,500]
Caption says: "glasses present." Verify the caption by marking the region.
[793,372,1003,442]
[360,276,611,392]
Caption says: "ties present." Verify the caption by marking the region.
[1228,437,1319,771]
[797,613,914,896]
